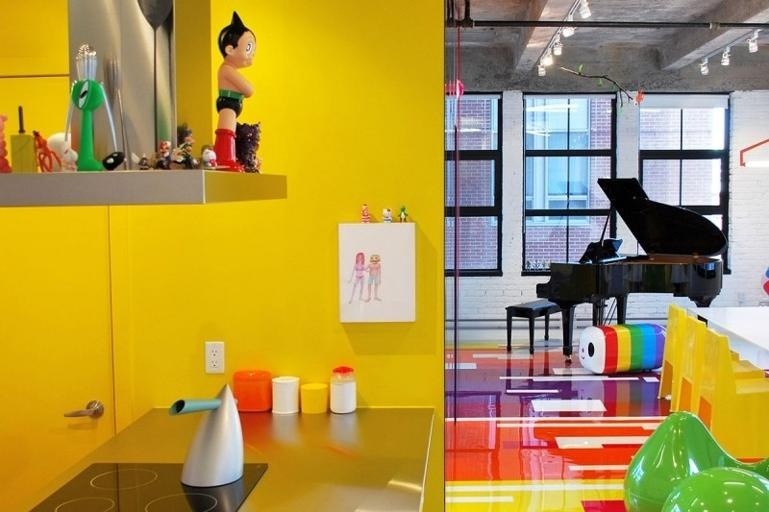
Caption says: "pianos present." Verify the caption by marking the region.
[536,178,732,362]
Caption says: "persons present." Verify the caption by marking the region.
[212,9,256,173]
[345,251,370,304]
[364,254,383,301]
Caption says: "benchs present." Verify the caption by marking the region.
[505,299,561,355]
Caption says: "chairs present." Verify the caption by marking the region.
[659,302,769,461]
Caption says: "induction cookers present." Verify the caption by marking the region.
[28,459,270,512]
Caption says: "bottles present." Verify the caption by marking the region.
[329,366,358,416]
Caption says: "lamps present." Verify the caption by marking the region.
[536,0,593,78]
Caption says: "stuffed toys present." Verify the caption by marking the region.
[360,203,371,225]
[397,204,408,223]
[381,208,393,223]
